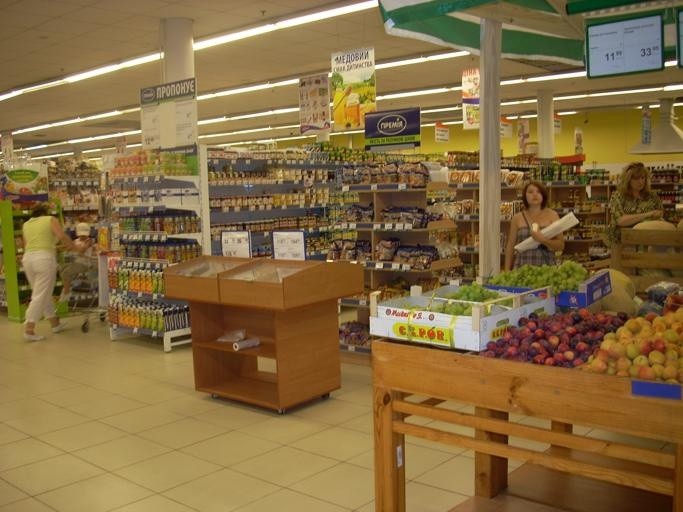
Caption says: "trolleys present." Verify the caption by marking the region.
[51,247,111,335]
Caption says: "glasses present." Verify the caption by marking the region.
[626,163,644,172]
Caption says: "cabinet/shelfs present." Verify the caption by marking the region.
[371,337,681,510]
[161,253,366,415]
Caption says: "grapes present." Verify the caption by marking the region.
[437,258,595,315]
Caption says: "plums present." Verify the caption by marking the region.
[478,306,683,385]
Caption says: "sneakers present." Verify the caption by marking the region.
[52,322,67,332]
[59,286,72,302]
[23,332,44,340]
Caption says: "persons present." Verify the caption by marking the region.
[607,161,681,277]
[501,183,567,272]
[18,202,83,341]
[56,223,94,305]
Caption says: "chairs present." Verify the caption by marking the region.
[2,139,681,369]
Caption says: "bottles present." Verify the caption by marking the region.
[120,243,201,264]
[646,163,683,183]
[120,216,201,234]
[657,190,683,204]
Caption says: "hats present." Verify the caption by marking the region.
[76,223,90,237]
